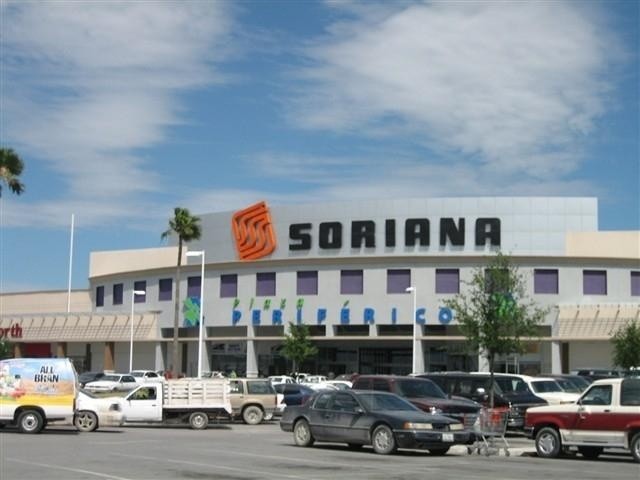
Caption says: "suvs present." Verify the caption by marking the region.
[352,375,491,454]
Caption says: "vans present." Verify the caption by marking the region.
[208,378,277,425]
[0,358,79,432]
[415,374,549,429]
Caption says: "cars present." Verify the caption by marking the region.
[263,372,357,420]
[84,373,141,394]
[280,389,469,455]
[470,369,640,405]
[128,370,165,381]
[79,372,105,388]
[47,388,131,432]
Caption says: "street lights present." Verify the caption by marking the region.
[185,250,205,379]
[129,290,146,374]
[405,286,417,375]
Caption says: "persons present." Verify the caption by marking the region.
[162,367,171,380]
[226,366,236,378]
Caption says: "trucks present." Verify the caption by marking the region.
[124,378,232,429]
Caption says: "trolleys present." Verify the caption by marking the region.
[467,401,513,457]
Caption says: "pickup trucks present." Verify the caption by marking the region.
[523,378,640,461]
